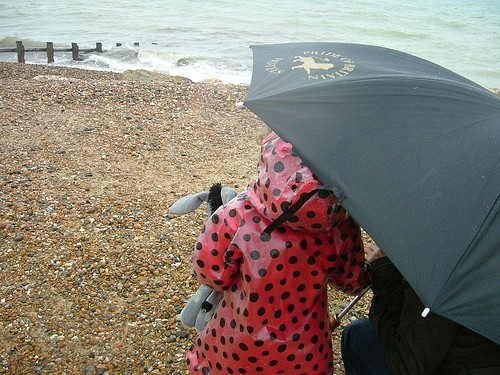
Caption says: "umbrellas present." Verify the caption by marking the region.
[243,43,500,345]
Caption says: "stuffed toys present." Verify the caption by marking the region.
[169,182,237,333]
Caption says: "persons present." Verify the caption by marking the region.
[186,130,367,375]
[339,242,500,375]
